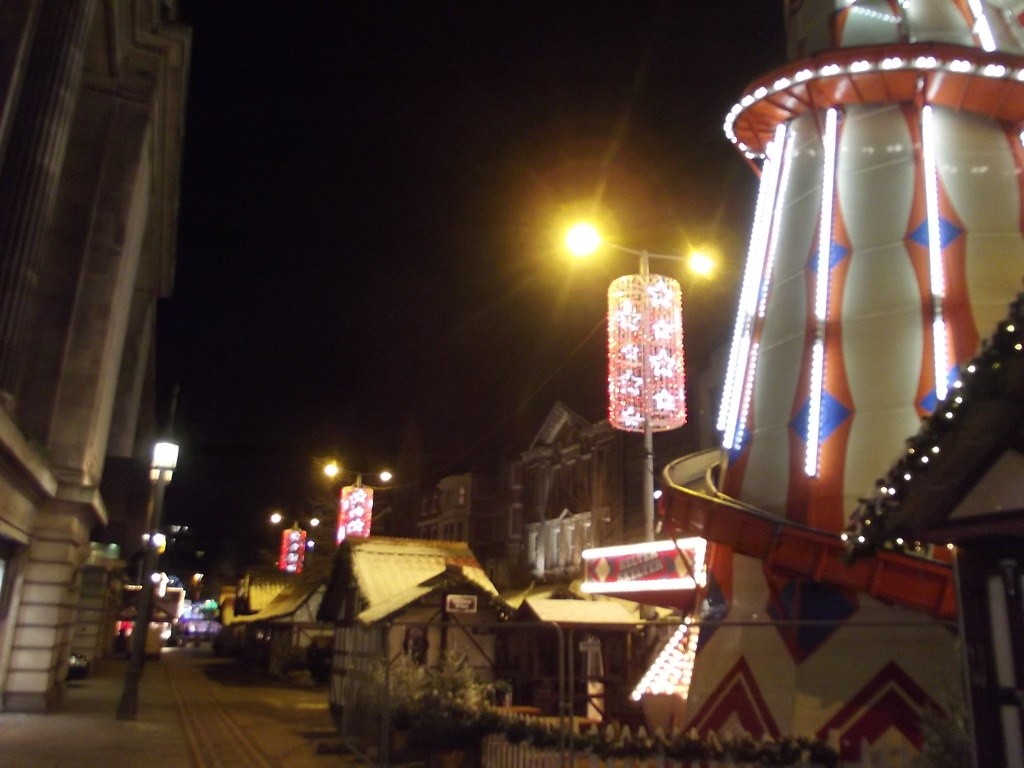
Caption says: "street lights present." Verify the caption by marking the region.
[323,461,396,488]
[569,222,720,642]
[112,438,179,724]
